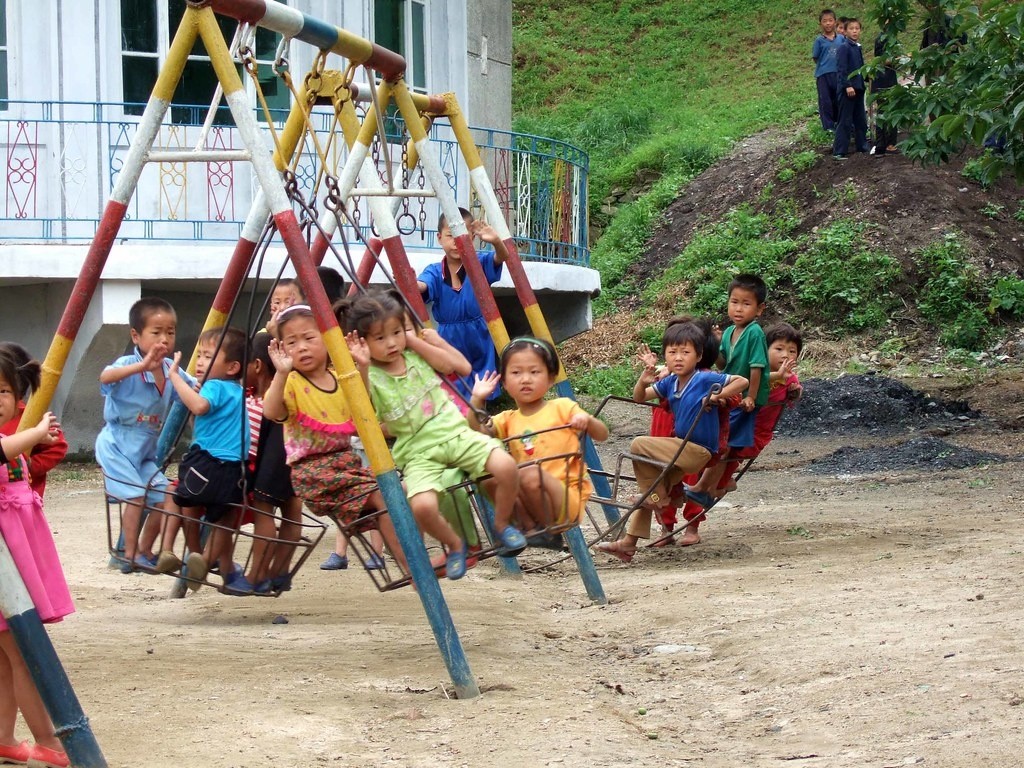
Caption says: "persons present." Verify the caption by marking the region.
[0,411,70,768]
[93,297,197,573]
[813,0,968,162]
[153,208,806,594]
[0,342,70,503]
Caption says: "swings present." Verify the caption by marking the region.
[102,49,600,599]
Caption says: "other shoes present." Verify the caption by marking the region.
[0,741,33,764]
[26,743,73,768]
[833,154,849,161]
[120,527,701,595]
[875,144,903,158]
[856,143,873,153]
[826,126,838,134]
